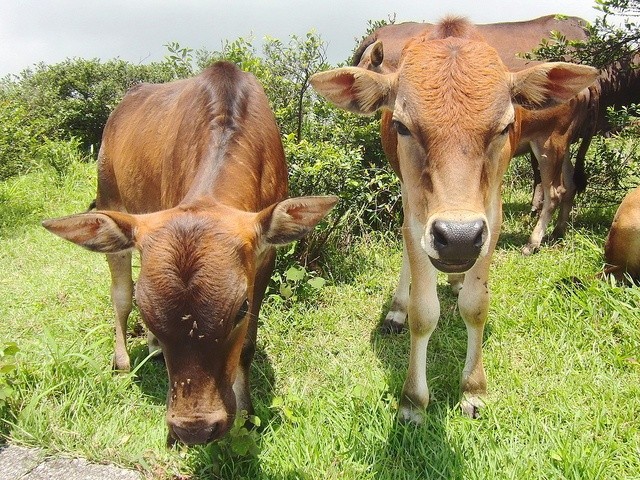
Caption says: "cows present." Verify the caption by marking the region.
[347,16,612,213]
[41,58,340,448]
[595,31,640,110]
[308,15,601,427]
[604,179,640,285]
[504,62,601,253]
